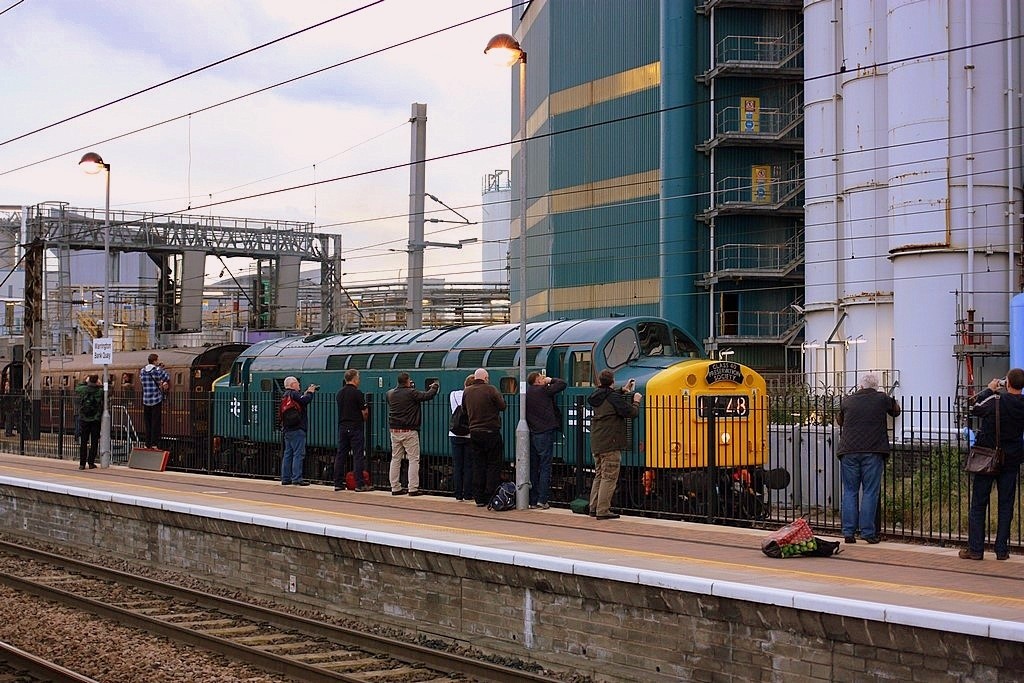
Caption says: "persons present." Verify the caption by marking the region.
[334,369,369,490]
[836,374,901,543]
[526,371,567,509]
[280,377,316,486]
[462,368,506,506]
[4,373,133,445]
[960,368,1024,560]
[450,374,475,500]
[74,375,104,470]
[385,372,439,496]
[139,354,170,450]
[587,368,642,520]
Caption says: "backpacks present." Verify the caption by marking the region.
[449,391,471,436]
[278,391,304,431]
[82,392,99,418]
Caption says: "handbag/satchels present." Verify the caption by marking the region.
[487,481,516,511]
[570,499,589,515]
[964,446,1005,474]
[761,513,817,559]
[159,382,168,392]
[799,537,844,559]
[346,471,370,490]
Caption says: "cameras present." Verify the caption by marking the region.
[309,384,321,391]
[996,377,1008,387]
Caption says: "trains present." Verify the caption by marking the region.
[0,312,791,530]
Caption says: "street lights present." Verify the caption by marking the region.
[480,33,532,510]
[77,152,111,468]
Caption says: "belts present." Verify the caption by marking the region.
[390,429,412,433]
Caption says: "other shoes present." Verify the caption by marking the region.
[335,486,345,491]
[997,553,1010,560]
[845,536,856,543]
[958,550,984,560]
[79,466,85,470]
[537,501,549,509]
[456,499,463,502]
[409,490,423,496]
[476,504,486,507]
[392,489,407,495]
[590,512,596,517]
[89,464,97,469]
[282,481,292,485]
[861,533,881,544]
[464,499,474,502]
[6,434,16,437]
[529,505,537,509]
[596,514,620,520]
[355,485,374,492]
[292,480,310,486]
